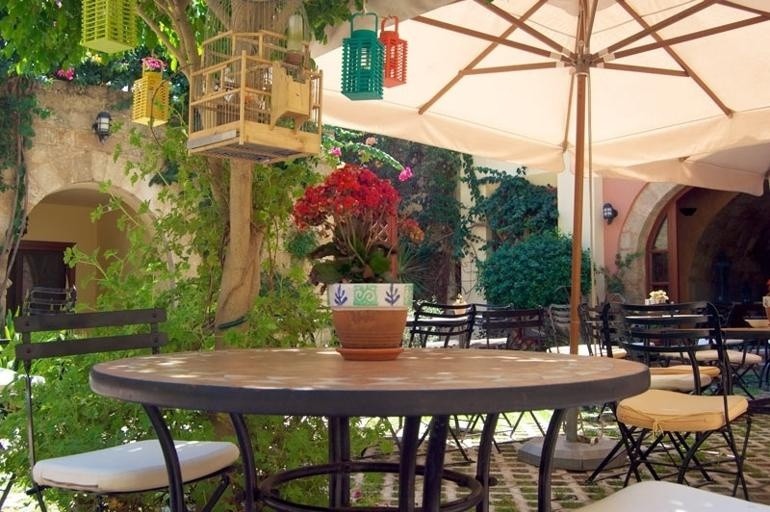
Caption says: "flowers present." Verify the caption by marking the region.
[766,279,770,296]
[292,163,425,296]
[648,290,669,301]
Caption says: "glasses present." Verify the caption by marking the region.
[92,110,112,143]
[602,203,618,225]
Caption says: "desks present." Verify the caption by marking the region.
[89,347,650,511]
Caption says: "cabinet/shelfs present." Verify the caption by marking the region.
[453,298,467,315]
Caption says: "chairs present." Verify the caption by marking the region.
[13,299,239,512]
[12,299,770,501]
[574,478,770,512]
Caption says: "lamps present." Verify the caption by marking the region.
[92,110,112,143]
[602,203,618,225]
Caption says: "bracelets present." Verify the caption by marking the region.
[744,319,768,328]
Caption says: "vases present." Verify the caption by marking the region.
[762,296,770,325]
[325,283,414,347]
[766,279,770,296]
[648,290,669,301]
[292,163,425,296]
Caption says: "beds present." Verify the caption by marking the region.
[308,0,770,440]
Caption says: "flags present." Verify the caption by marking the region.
[325,283,414,347]
[762,296,770,325]
